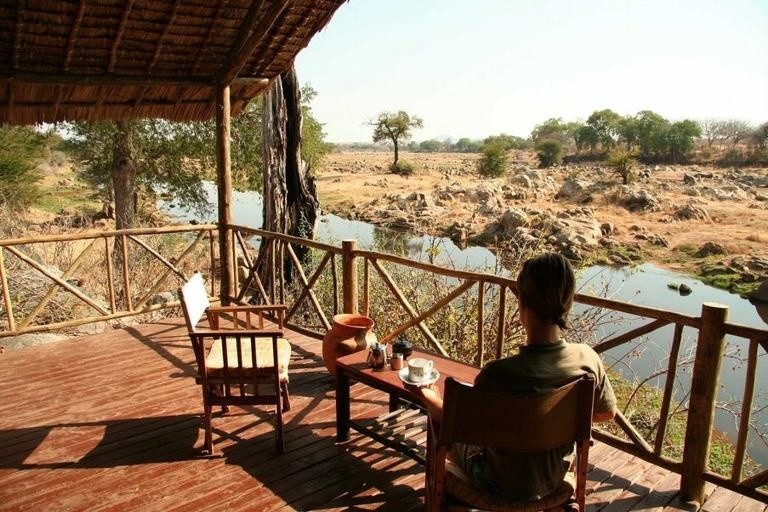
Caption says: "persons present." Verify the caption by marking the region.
[403,253,617,511]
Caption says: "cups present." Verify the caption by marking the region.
[407,357,434,384]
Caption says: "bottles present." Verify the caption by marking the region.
[391,352,404,370]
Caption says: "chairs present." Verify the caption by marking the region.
[419,373,594,511]
[178,270,292,455]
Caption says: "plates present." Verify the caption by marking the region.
[397,365,440,387]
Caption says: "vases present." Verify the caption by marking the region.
[322,313,378,386]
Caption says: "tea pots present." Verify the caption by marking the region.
[365,341,390,370]
